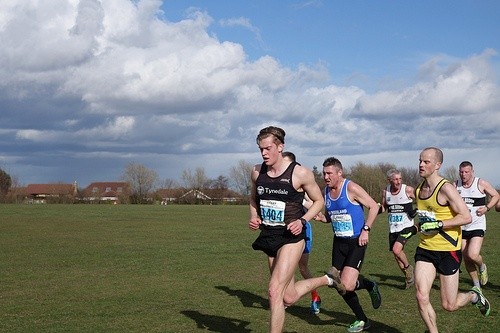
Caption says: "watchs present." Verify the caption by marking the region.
[437,220,444,229]
[362,225,371,232]
[299,217,307,225]
[485,205,490,211]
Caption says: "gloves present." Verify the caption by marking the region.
[419,220,442,233]
[399,225,418,239]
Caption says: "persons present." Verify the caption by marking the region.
[247,127,346,333]
[281,152,500,333]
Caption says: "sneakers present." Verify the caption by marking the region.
[326,267,347,297]
[479,264,489,285]
[404,281,415,289]
[311,296,321,314]
[347,319,370,332]
[368,280,381,309]
[404,264,414,282]
[468,286,491,317]
